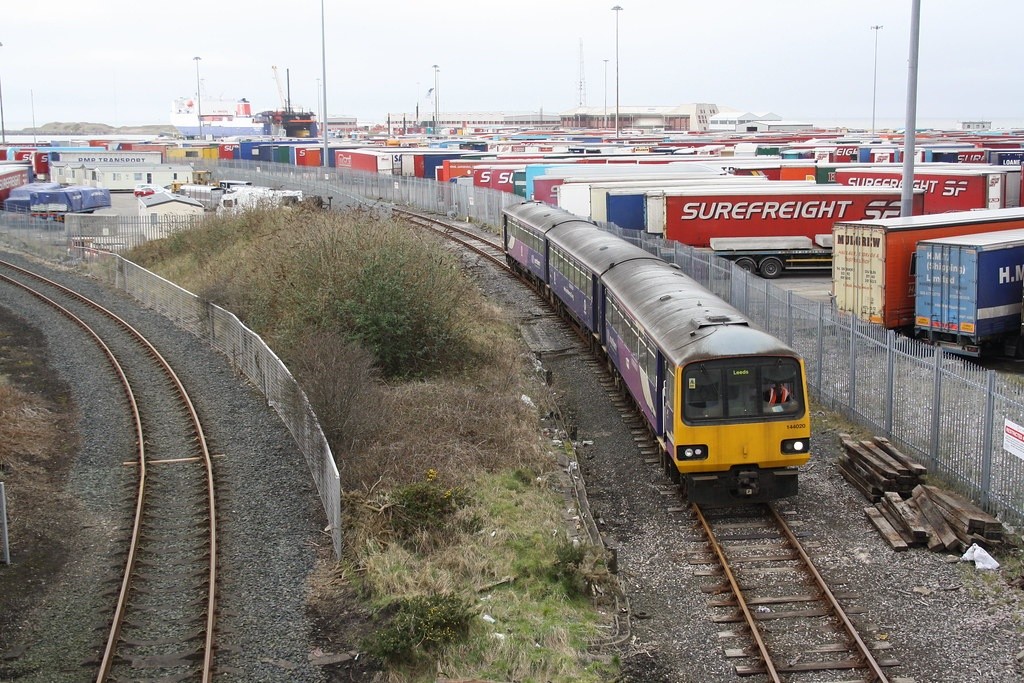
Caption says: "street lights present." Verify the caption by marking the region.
[192,56,202,140]
[610,5,623,137]
[431,64,441,128]
[603,59,610,131]
[870,24,883,135]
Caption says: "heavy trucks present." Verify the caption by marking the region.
[0,128,1024,367]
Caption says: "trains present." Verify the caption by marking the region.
[499,199,811,507]
